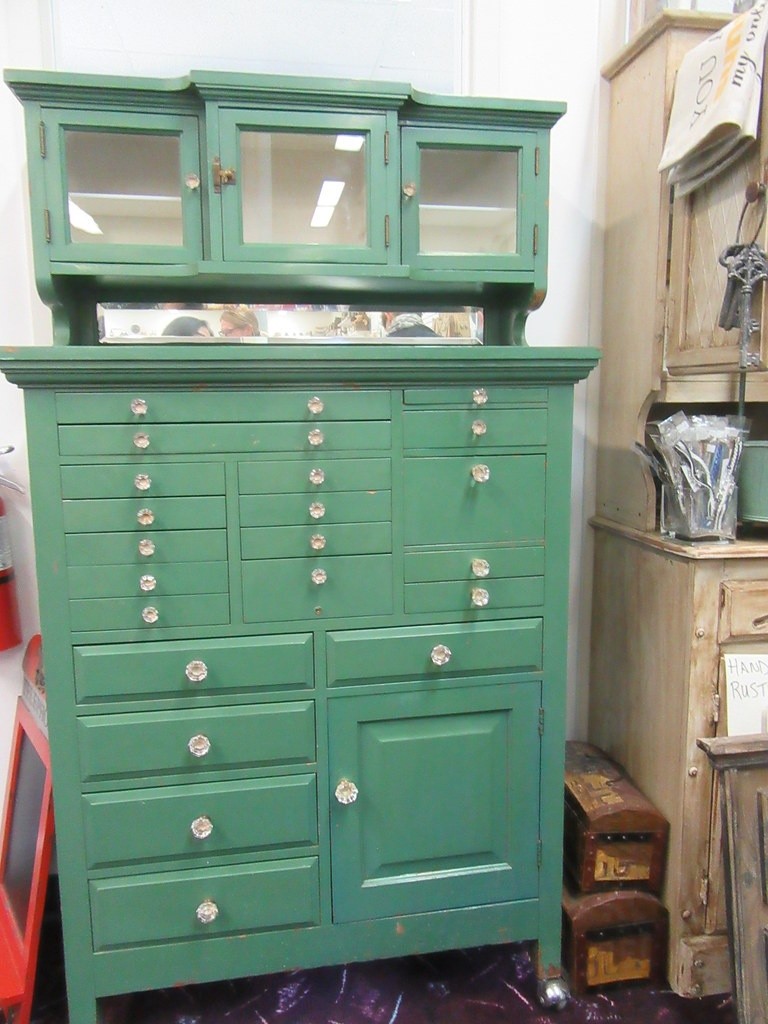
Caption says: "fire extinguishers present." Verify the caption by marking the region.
[0,447,25,655]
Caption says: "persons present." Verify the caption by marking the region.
[162,317,214,338]
[381,311,438,337]
[218,307,259,338]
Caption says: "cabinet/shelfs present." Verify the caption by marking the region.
[601,13,768,1003]
[2,68,601,1024]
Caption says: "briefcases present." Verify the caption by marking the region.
[561,881,668,993]
[563,740,671,892]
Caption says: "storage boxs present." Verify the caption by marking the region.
[564,741,671,997]
